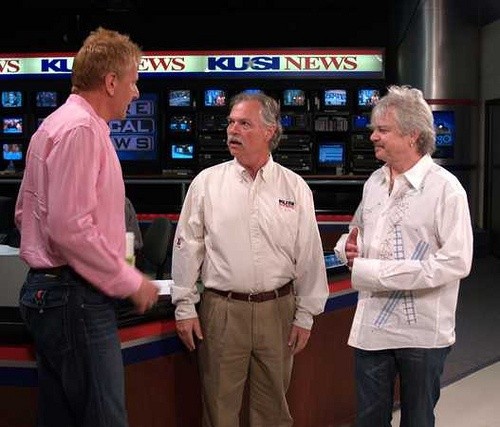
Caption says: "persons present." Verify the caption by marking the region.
[333,84,473,427]
[16,26,160,427]
[171,92,330,427]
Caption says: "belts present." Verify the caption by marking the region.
[210,280,294,304]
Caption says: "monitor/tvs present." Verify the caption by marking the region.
[0,85,383,169]
[431,108,458,159]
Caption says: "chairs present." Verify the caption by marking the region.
[139,216,174,280]
